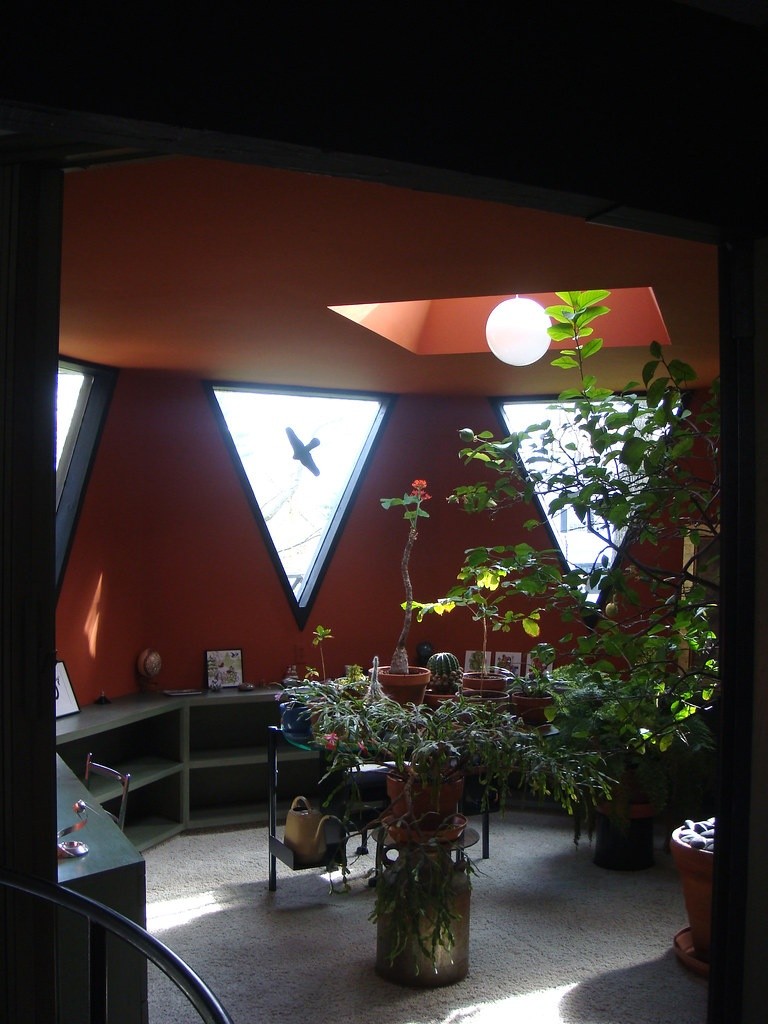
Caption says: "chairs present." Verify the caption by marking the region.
[0,871,231,1024]
[84,751,132,832]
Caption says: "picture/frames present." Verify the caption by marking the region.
[56,661,82,720]
[205,649,243,691]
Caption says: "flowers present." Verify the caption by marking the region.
[379,479,433,674]
[276,623,621,978]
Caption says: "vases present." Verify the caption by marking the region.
[381,769,469,843]
[368,664,431,714]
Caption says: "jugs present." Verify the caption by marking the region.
[284,796,331,863]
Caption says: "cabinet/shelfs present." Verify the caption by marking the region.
[184,685,323,829]
[57,753,150,1024]
[56,694,186,852]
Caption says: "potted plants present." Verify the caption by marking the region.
[424,289,716,976]
